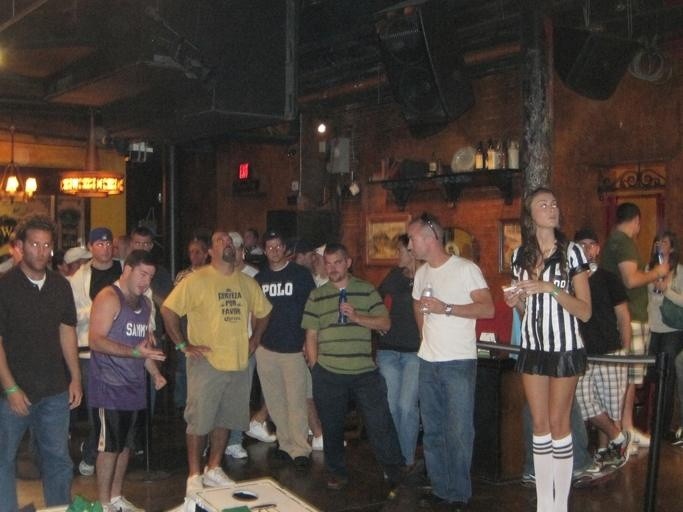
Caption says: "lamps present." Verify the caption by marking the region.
[0,126,41,207]
[59,108,124,200]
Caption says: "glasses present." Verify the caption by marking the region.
[421,213,438,240]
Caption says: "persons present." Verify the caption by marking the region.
[0,214,683,510]
[501,185,591,510]
[603,203,669,451]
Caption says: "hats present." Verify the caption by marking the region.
[574,229,597,243]
[90,228,112,243]
[64,247,92,265]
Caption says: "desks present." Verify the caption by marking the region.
[187,475,323,512]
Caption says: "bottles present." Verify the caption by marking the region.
[586,261,598,279]
[654,244,667,268]
[428,150,438,176]
[475,135,527,173]
[414,281,433,332]
[652,278,666,295]
[336,287,348,325]
[435,156,443,176]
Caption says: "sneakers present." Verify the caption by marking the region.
[611,430,633,468]
[572,462,616,488]
[113,497,145,512]
[595,450,610,464]
[226,421,347,489]
[521,472,535,486]
[186,475,202,495]
[78,443,95,475]
[203,466,234,487]
[101,503,118,512]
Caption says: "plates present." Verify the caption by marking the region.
[450,145,477,174]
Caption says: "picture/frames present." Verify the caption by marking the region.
[363,213,412,265]
[498,217,524,273]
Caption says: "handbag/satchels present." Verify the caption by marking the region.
[660,297,683,329]
